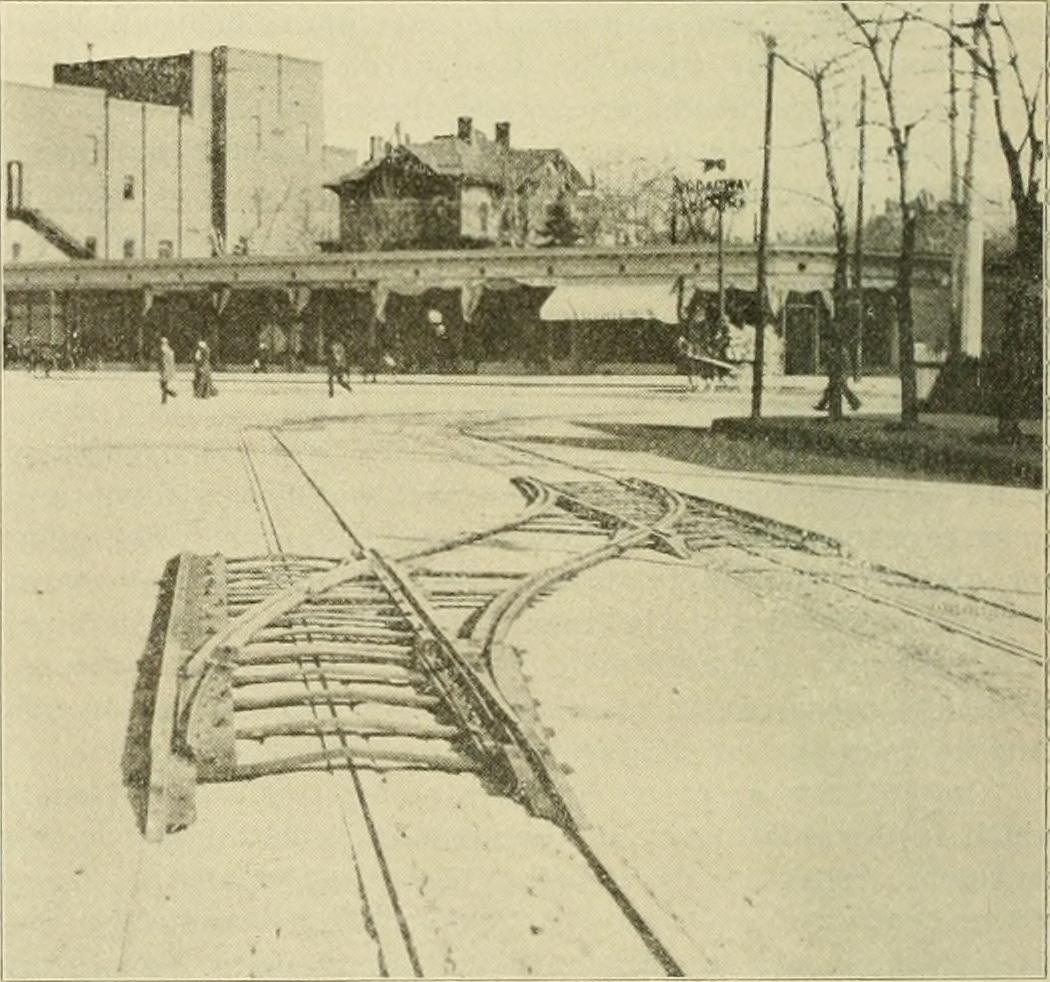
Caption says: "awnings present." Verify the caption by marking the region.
[539,282,697,325]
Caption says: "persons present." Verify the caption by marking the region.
[326,338,355,400]
[191,340,211,400]
[157,338,177,406]
[811,345,861,411]
[252,343,272,374]
